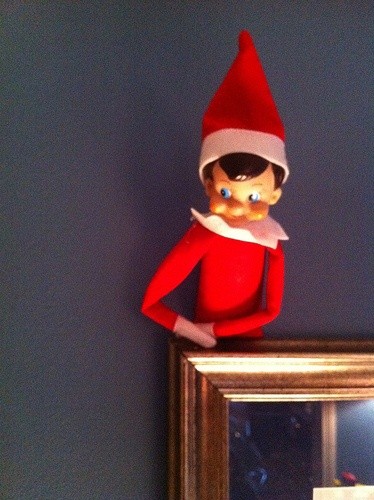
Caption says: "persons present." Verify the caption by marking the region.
[140,23,288,348]
